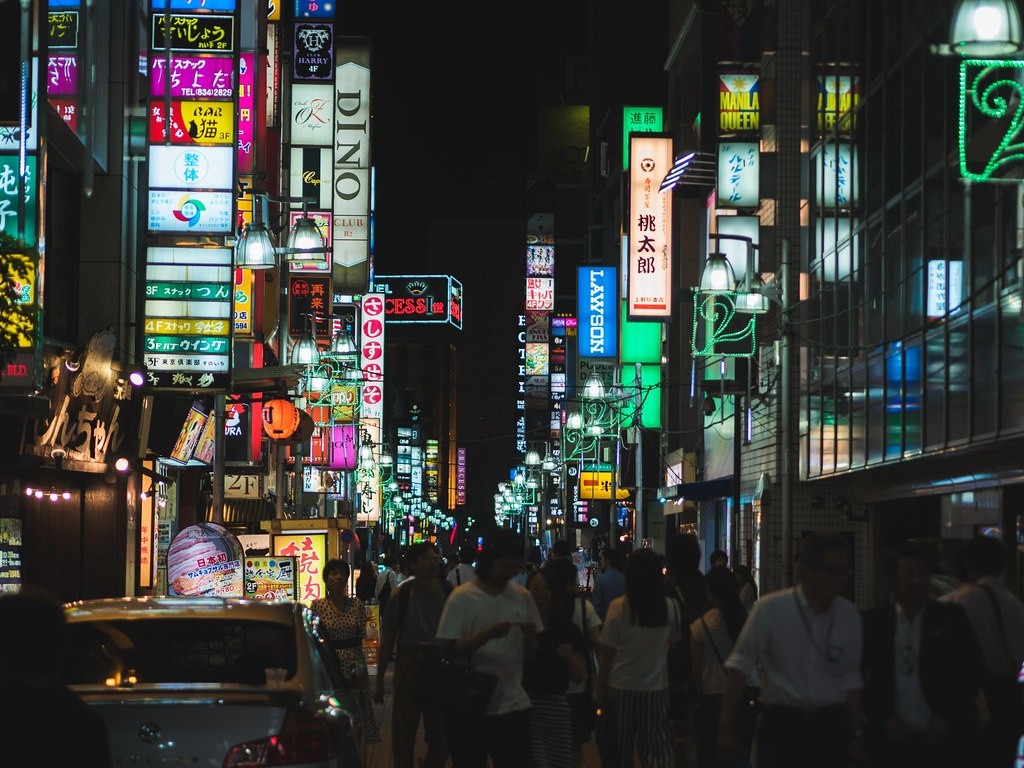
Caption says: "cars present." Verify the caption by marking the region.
[44,597,368,768]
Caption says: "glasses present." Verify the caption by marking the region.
[418,553,440,562]
[899,645,915,675]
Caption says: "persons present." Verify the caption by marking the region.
[354,519,1024,768]
[2,586,114,768]
[308,558,383,746]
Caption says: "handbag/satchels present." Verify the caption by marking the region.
[409,650,500,724]
